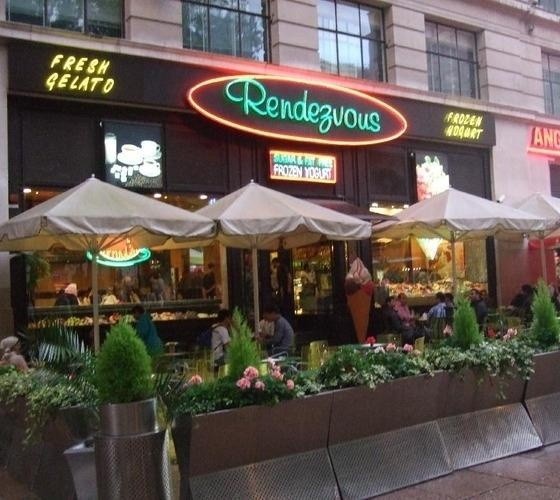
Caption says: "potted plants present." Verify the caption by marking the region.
[96,321,157,435]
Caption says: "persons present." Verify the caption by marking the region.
[424,292,457,343]
[378,293,423,347]
[208,305,294,366]
[53,270,163,306]
[463,289,490,335]
[2,337,28,370]
[382,265,441,286]
[131,305,162,356]
[511,284,559,328]
[194,262,216,299]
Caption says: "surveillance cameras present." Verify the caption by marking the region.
[497,194,506,203]
